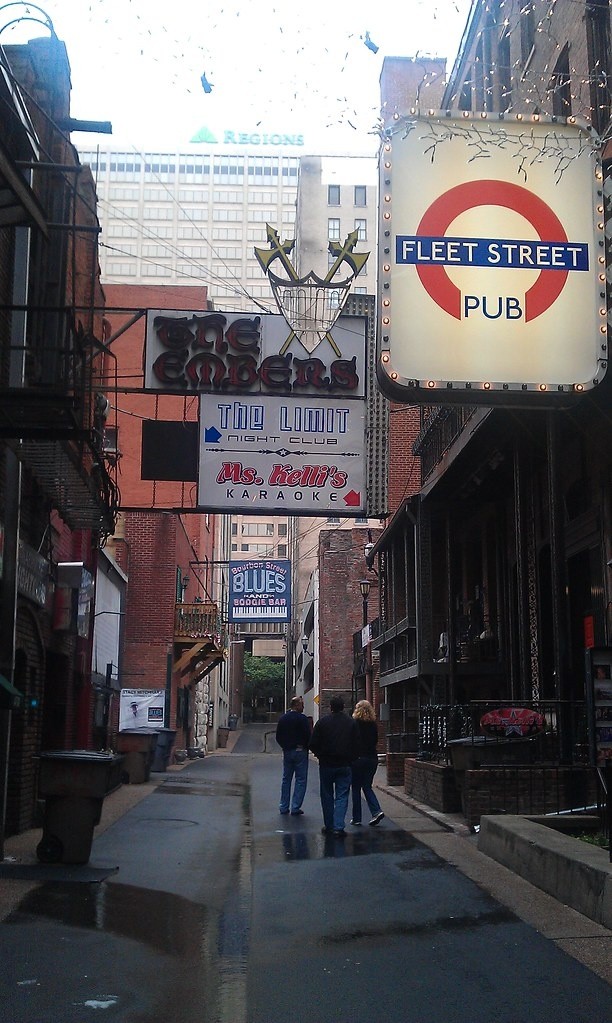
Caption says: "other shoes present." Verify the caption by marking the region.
[281,808,290,814]
[323,827,345,836]
[291,809,304,816]
[350,819,361,825]
[369,812,384,826]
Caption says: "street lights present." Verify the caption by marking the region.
[269,698,273,723]
[360,577,371,673]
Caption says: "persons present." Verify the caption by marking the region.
[309,695,359,837]
[350,700,385,826]
[276,697,311,816]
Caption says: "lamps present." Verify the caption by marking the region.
[364,542,378,577]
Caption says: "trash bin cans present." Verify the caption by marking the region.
[227,714,239,731]
[114,726,159,785]
[445,734,539,818]
[139,725,178,772]
[35,749,115,876]
[217,725,231,748]
